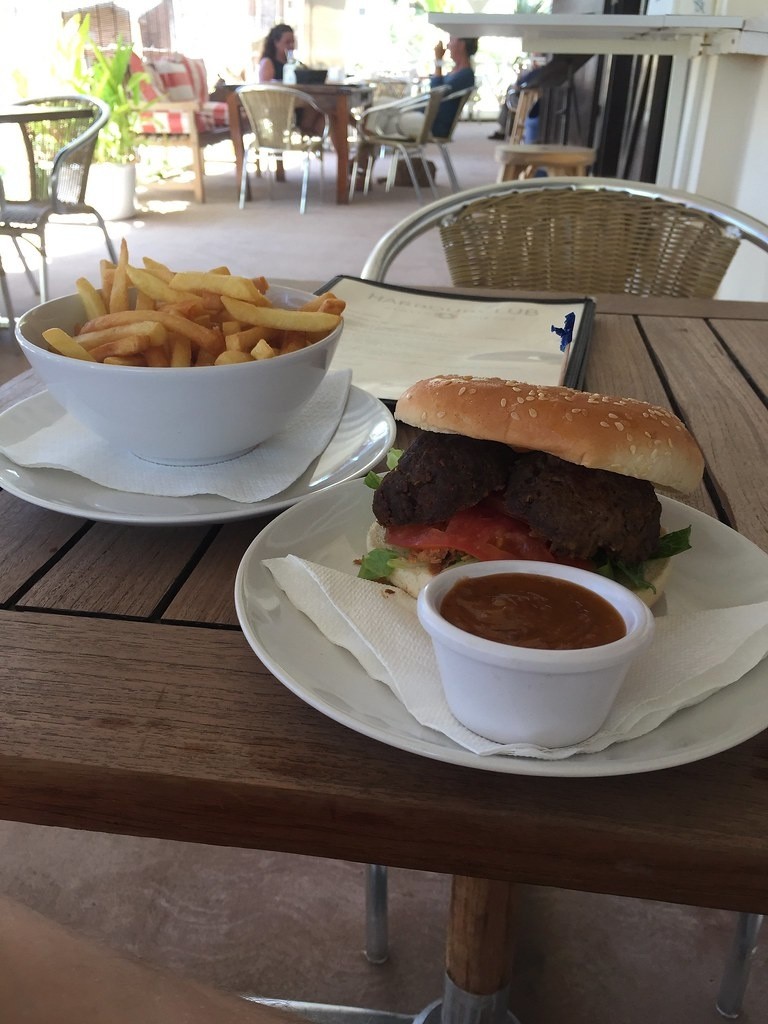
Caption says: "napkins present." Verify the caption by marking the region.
[261,554,768,761]
[0,366,353,503]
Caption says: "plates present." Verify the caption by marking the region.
[0,390,397,526]
[235,469,768,774]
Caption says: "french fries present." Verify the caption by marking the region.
[41,237,346,367]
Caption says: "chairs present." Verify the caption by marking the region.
[360,175,768,1024]
[235,84,330,214]
[348,85,451,207]
[384,86,475,194]
[0,92,117,304]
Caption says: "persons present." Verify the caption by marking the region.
[259,23,325,135]
[488,52,547,140]
[347,36,478,192]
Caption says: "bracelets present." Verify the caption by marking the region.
[434,58,444,65]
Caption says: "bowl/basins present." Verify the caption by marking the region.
[15,283,344,466]
[417,560,652,746]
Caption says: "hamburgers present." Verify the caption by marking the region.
[353,374,705,608]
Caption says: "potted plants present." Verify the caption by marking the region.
[67,34,154,221]
[13,11,98,225]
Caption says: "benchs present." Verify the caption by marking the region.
[84,47,250,205]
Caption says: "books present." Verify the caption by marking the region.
[313,274,598,406]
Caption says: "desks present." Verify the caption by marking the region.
[0,105,95,356]
[220,83,376,206]
[0,278,768,1024]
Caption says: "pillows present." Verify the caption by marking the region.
[104,51,170,102]
[154,57,210,102]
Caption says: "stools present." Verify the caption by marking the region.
[492,144,595,183]
